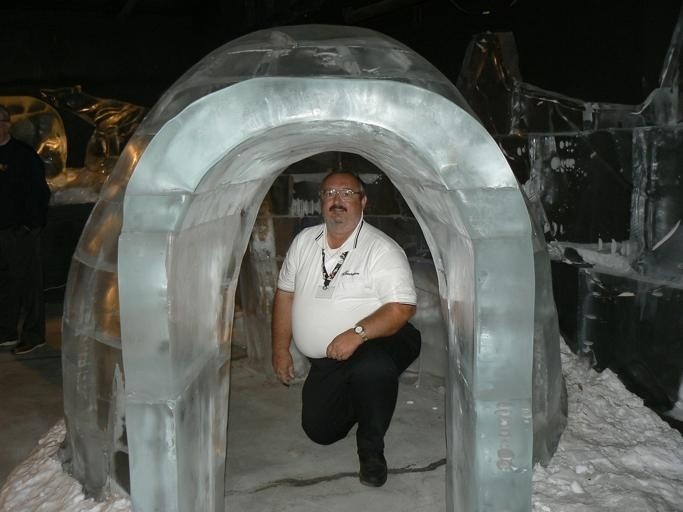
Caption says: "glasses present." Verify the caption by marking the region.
[322,189,362,199]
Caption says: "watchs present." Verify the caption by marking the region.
[351,325,368,341]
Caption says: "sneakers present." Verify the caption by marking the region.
[0,330,47,355]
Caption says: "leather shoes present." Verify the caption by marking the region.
[359,447,388,488]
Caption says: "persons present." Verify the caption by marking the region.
[0,105,52,355]
[272,171,422,488]
